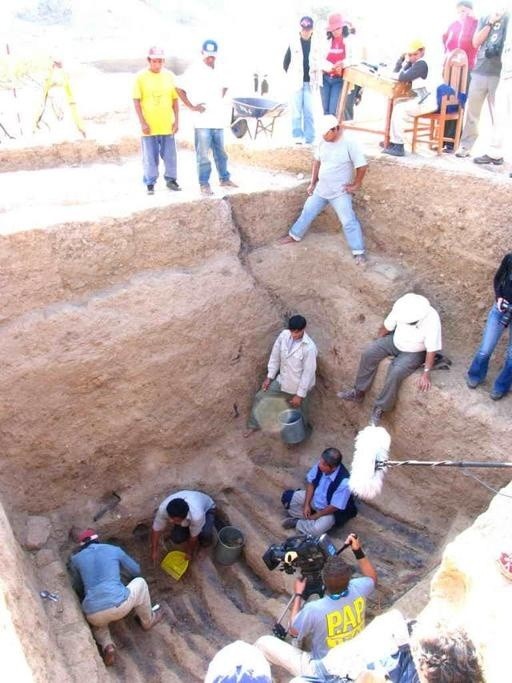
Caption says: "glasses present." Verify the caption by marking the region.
[325,589,349,600]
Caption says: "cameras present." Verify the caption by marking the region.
[263,533,360,576]
[497,300,512,327]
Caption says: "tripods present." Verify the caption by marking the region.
[273,574,327,641]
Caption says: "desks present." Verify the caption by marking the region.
[336,61,439,149]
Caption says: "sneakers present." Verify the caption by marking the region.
[454,146,471,157]
[282,518,298,529]
[387,144,405,156]
[336,389,365,402]
[473,154,505,164]
[166,180,182,191]
[151,608,165,627]
[379,141,385,148]
[147,185,155,195]
[497,553,512,581]
[105,646,114,667]
[219,181,239,188]
[442,145,453,153]
[305,143,312,148]
[490,390,503,401]
[370,407,384,426]
[431,146,439,151]
[295,137,303,144]
[467,379,479,389]
[200,184,214,195]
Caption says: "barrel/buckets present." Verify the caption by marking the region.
[217,525,245,566]
[279,410,307,444]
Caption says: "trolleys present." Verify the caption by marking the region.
[229,98,290,143]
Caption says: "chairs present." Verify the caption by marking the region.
[402,47,469,152]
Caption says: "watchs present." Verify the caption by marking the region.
[423,367,433,372]
[294,592,303,596]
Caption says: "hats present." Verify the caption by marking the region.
[317,114,339,135]
[202,40,218,58]
[77,530,98,546]
[300,16,314,28]
[392,293,429,324]
[405,40,425,52]
[326,14,345,31]
[147,45,166,59]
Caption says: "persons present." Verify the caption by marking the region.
[284,15,323,149]
[341,20,363,121]
[151,489,218,569]
[131,46,182,193]
[472,10,512,179]
[379,39,439,155]
[243,314,319,450]
[454,6,509,157]
[430,0,480,152]
[355,622,512,683]
[308,11,365,116]
[70,530,166,666]
[253,532,377,677]
[174,39,239,194]
[280,447,359,537]
[276,114,370,268]
[336,293,442,426]
[466,252,512,400]
